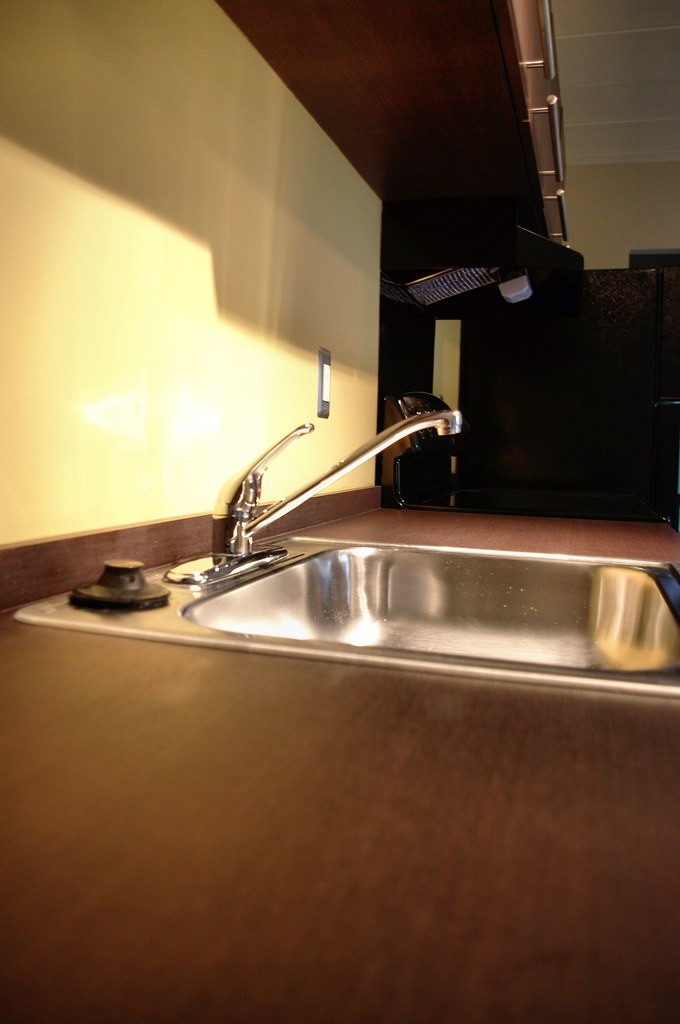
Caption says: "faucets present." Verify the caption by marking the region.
[212,409,464,556]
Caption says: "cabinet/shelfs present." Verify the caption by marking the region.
[213,0,570,248]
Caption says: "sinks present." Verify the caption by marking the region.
[177,544,679,673]
